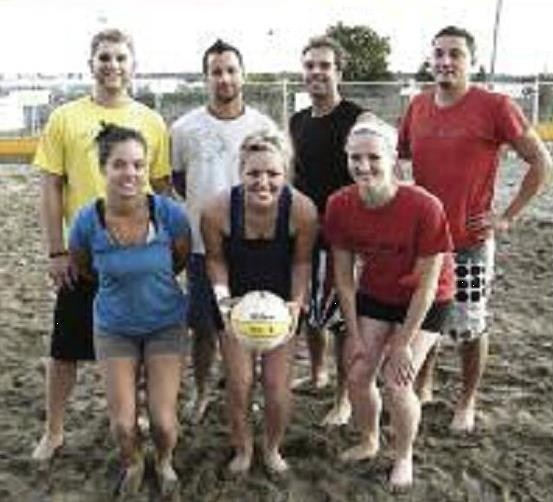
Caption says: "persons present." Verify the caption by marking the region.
[28,28,174,463]
[285,36,374,428]
[323,111,456,496]
[392,24,551,439]
[66,118,193,496]
[199,130,320,473]
[166,35,290,429]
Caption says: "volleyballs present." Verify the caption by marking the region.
[230,290,294,352]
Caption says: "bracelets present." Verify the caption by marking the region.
[47,250,68,257]
[212,285,231,314]
